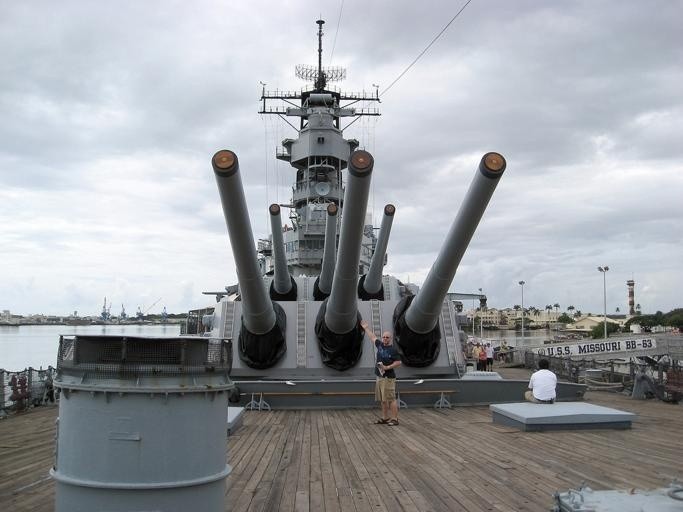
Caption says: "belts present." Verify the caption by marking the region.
[537,399,551,402]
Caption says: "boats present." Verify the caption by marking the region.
[541,332,583,345]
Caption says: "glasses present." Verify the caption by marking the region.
[383,336,389,338]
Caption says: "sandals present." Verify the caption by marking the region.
[374,418,391,424]
[388,418,398,425]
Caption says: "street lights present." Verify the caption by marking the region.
[597,264,609,340]
[476,287,482,339]
[518,281,526,336]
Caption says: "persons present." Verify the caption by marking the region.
[524,358,556,403]
[358,318,403,427]
[467,339,508,372]
